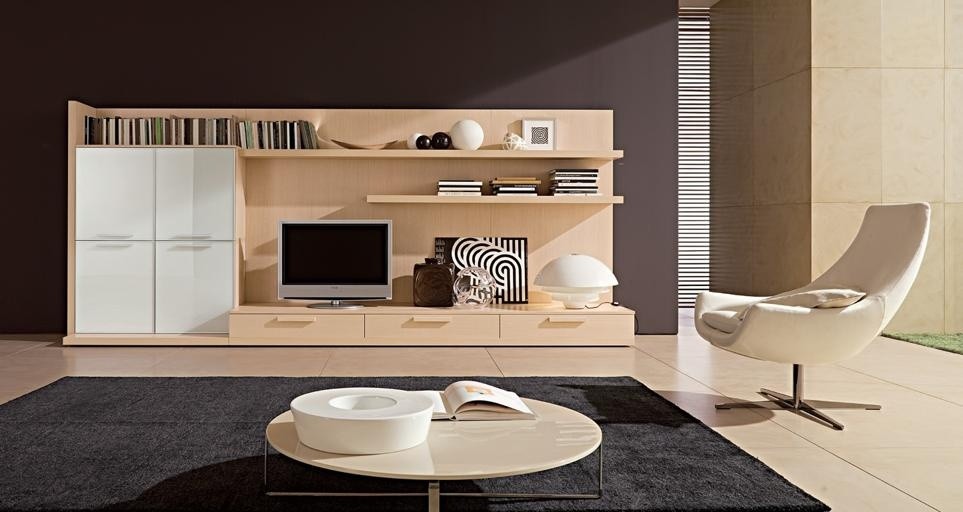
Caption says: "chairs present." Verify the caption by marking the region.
[694,201,932,431]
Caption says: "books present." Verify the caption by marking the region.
[84,114,319,150]
[408,380,537,420]
[437,168,601,196]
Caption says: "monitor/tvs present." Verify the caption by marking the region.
[277,220,392,309]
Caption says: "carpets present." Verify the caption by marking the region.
[0,375,833,512]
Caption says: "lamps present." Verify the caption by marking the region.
[533,254,619,310]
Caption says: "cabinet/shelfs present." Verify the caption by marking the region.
[63,101,636,347]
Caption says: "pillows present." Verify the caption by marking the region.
[738,287,867,321]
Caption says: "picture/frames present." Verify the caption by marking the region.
[521,116,558,152]
[521,117,555,149]
